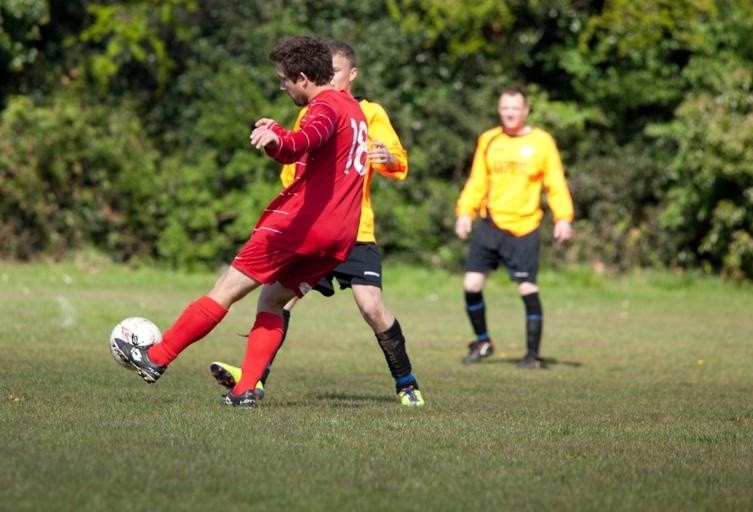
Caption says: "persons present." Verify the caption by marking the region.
[115,38,368,407]
[455,86,575,370]
[211,42,425,408]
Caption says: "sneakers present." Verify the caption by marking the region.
[522,352,543,369]
[461,339,494,365]
[107,337,167,385]
[210,362,264,409]
[394,379,425,409]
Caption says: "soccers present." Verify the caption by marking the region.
[110,317,163,369]
